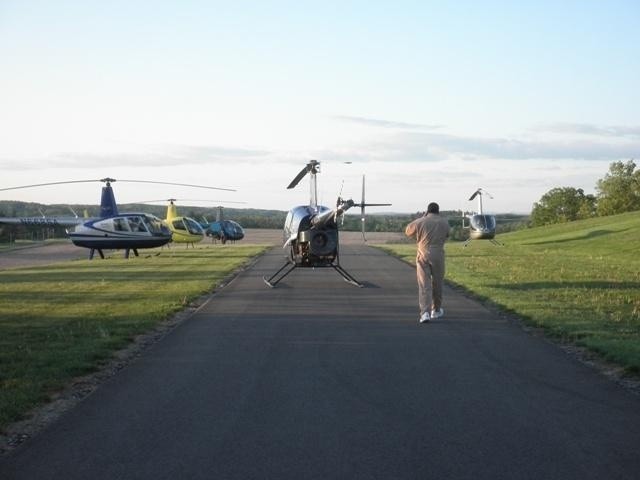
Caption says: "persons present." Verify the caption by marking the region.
[405,201,450,324]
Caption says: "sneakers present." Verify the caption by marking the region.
[419,308,444,323]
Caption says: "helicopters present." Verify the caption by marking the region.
[462,187,504,248]
[0,177,245,262]
[262,159,392,289]
